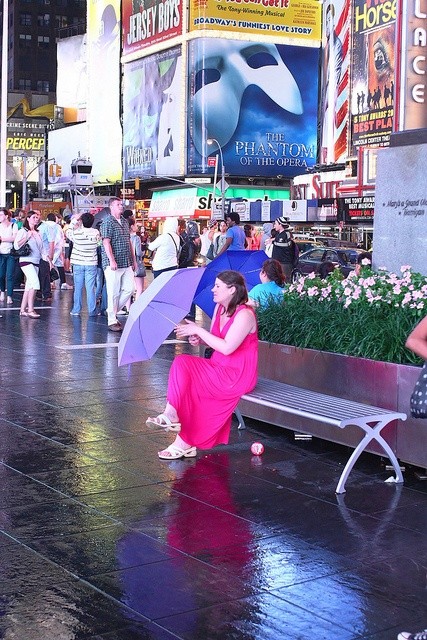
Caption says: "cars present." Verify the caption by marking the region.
[292,234,357,254]
[291,248,370,284]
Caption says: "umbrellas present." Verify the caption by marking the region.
[192,250,270,320]
[117,267,206,383]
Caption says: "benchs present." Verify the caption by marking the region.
[233,376,407,495]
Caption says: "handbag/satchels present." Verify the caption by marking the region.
[206,235,220,260]
[409,362,427,419]
[10,244,32,256]
[134,260,146,277]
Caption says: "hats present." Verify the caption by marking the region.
[186,221,199,238]
[276,216,289,226]
[207,218,217,228]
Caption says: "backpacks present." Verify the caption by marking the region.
[178,231,196,267]
[289,232,299,260]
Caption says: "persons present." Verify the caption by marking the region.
[146,270,258,460]
[346,252,371,278]
[251,225,259,250]
[137,226,147,251]
[243,225,254,250]
[15,210,26,231]
[146,218,180,279]
[213,222,227,257]
[260,223,273,258]
[264,229,279,258]
[176,220,190,270]
[247,259,286,312]
[67,213,102,318]
[213,213,249,257]
[0,209,19,304]
[199,227,210,255]
[63,204,71,217]
[205,219,218,260]
[405,315,427,359]
[33,209,54,302]
[44,214,74,290]
[99,197,135,331]
[62,214,73,274]
[14,211,53,318]
[10,210,17,224]
[272,217,294,276]
[123,210,136,219]
[126,220,145,314]
[55,213,64,230]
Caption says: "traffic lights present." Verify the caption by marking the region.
[56,164,61,177]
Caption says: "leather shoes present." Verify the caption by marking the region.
[108,319,124,331]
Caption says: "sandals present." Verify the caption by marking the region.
[0,292,4,301]
[7,296,13,303]
[158,446,197,460]
[146,414,181,433]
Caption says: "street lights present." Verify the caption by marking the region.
[207,138,224,223]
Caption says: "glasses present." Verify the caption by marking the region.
[32,217,38,219]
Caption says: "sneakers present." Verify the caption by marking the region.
[50,283,55,289]
[36,292,42,301]
[28,311,40,318]
[20,310,28,317]
[69,311,80,316]
[43,297,52,302]
[61,283,73,290]
